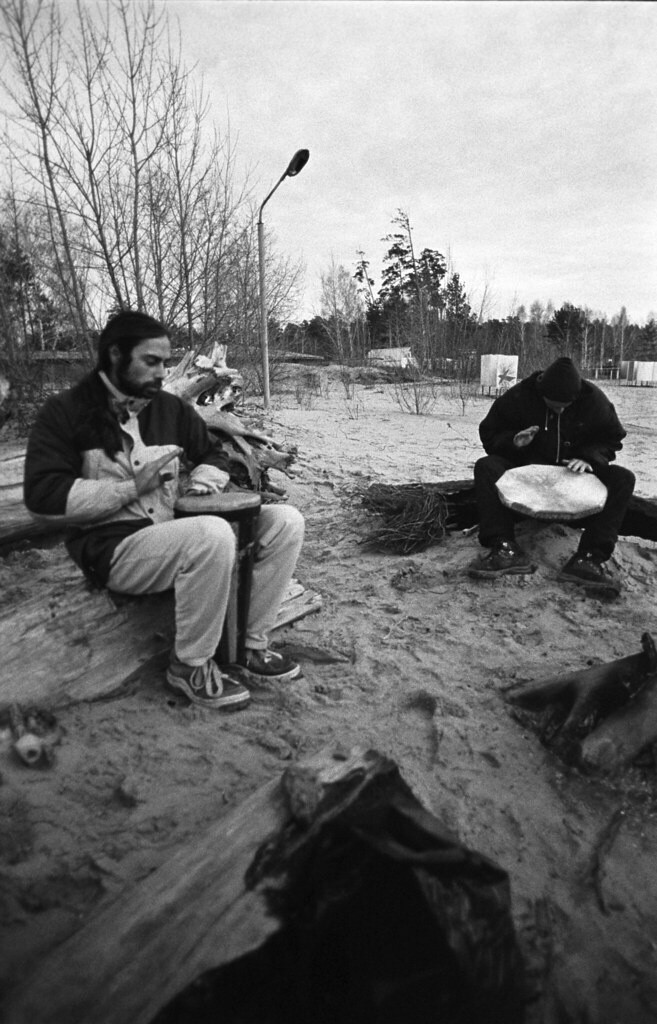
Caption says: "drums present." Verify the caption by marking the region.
[171,491,265,684]
[494,462,609,522]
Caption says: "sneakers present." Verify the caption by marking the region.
[558,553,619,598]
[214,648,299,680]
[466,540,533,579]
[163,659,250,712]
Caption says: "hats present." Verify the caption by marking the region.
[535,357,593,403]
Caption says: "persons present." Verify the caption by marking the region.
[467,357,636,586]
[23,310,306,711]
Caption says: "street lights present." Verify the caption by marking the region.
[257,148,309,408]
